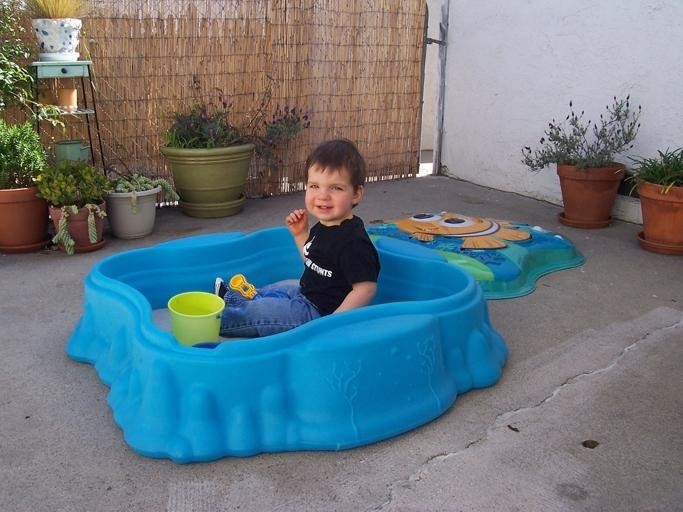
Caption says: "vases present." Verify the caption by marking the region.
[58,87,78,112]
[157,144,256,218]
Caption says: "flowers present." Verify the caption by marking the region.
[157,68,312,179]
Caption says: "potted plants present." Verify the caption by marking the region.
[0,105,181,252]
[24,0,92,61]
[519,94,642,229]
[614,145,683,255]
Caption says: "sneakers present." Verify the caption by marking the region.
[215,277,229,299]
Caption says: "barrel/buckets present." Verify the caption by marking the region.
[169,291,224,344]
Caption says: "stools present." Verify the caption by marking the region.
[28,60,109,180]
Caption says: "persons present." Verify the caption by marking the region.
[214,139,381,337]
